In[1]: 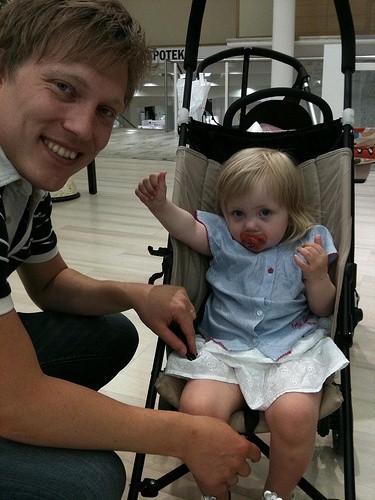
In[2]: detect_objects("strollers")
[127,0,364,500]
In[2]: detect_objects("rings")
[190,306,195,314]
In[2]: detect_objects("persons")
[0,0,262,500]
[134,149,351,500]
